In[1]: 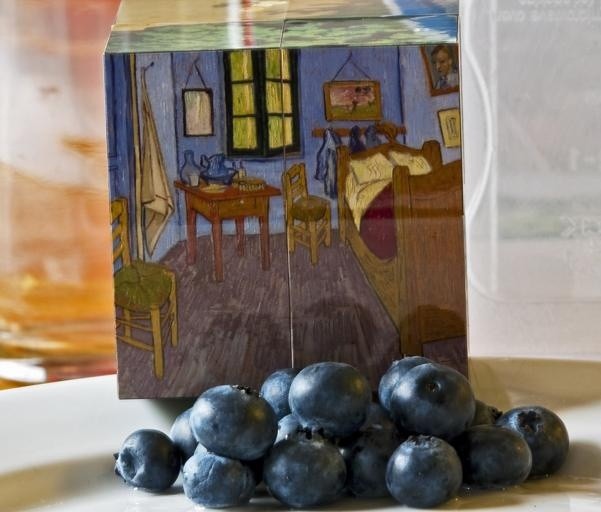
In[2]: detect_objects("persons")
[431,44,459,90]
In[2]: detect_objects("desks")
[2,356,601,512]
